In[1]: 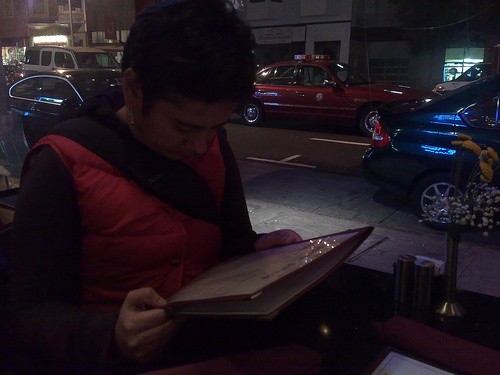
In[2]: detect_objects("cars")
[432,62,494,99]
[0,72,121,129]
[358,72,499,231]
[230,55,422,138]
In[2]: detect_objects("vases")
[425,221,480,317]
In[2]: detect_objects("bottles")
[395,253,416,304]
[414,260,434,309]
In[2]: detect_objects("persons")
[8,0,304,375]
[86,57,93,65]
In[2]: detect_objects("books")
[163,227,374,319]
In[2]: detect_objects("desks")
[139,262,500,375]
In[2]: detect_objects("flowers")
[418,131,500,241]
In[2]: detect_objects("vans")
[23,46,121,79]
